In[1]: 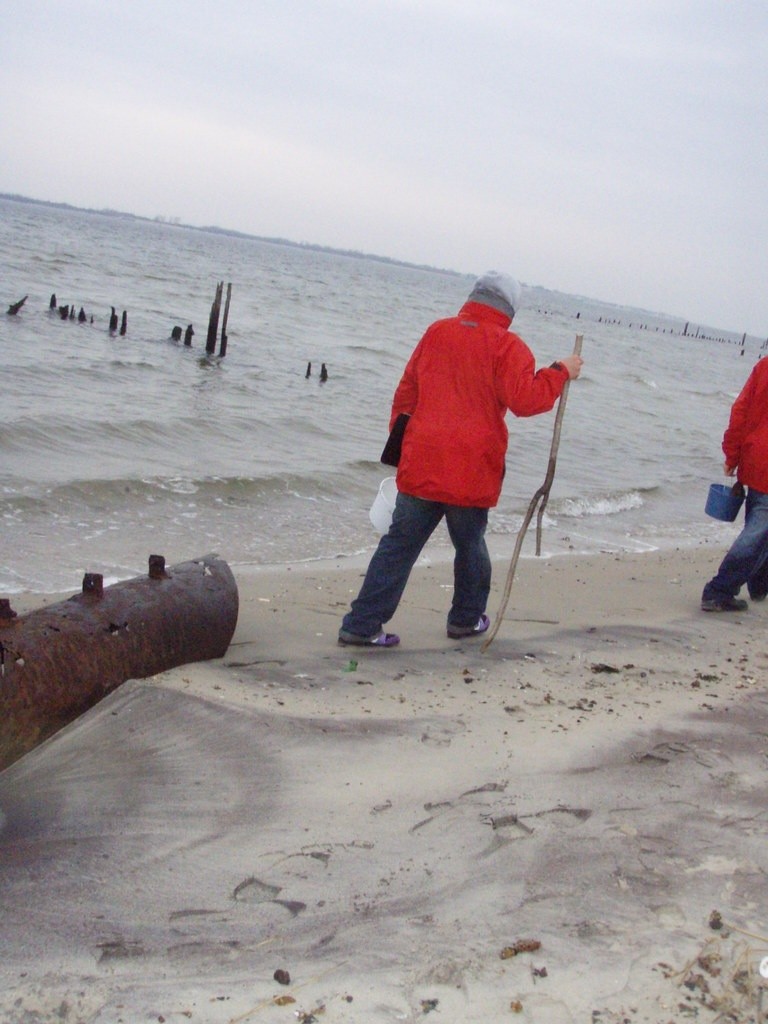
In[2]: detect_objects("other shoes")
[338,627,400,647]
[701,595,748,613]
[751,590,767,601]
[447,614,490,640]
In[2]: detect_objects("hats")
[466,270,522,313]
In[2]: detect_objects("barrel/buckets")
[369,475,398,533]
[704,471,746,523]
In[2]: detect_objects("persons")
[700,355,768,612]
[339,272,584,646]
